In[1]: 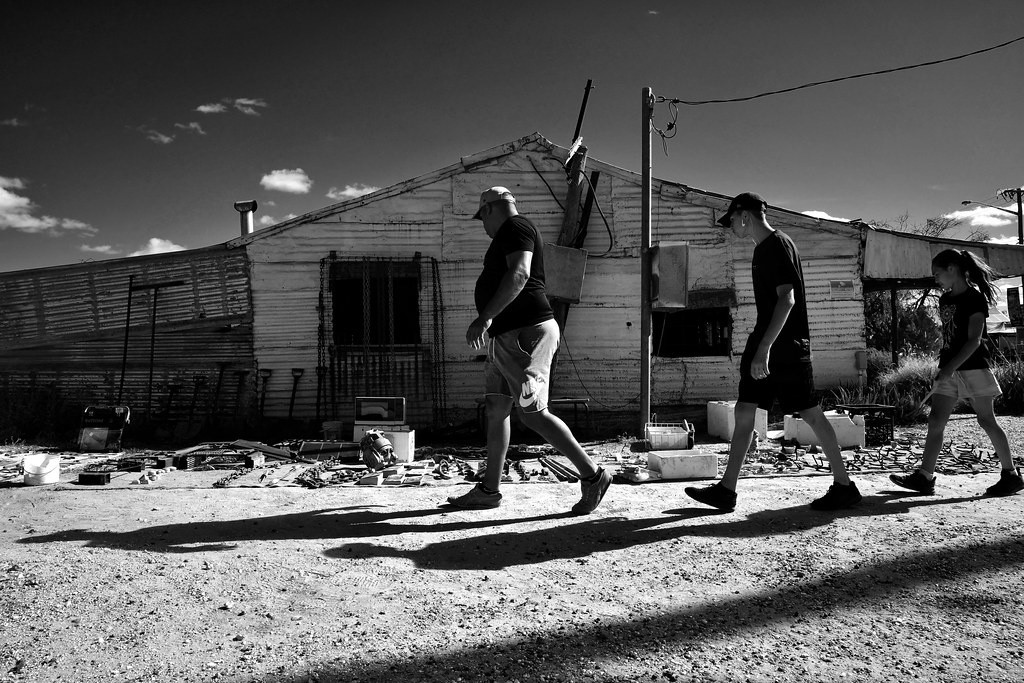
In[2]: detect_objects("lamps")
[235,199,256,236]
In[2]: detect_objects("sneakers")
[889,469,936,494]
[684,483,737,511]
[572,469,613,516]
[447,483,502,508]
[986,468,1024,496]
[809,481,862,511]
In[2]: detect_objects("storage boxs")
[646,426,689,449]
[354,425,416,463]
[707,401,767,442]
[648,450,718,480]
[835,403,897,446]
[783,413,865,448]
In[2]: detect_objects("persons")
[889,248,1024,496]
[447,185,613,514]
[684,191,863,512]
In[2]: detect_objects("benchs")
[475,398,592,438]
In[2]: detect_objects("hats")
[471,186,517,220]
[717,192,768,228]
